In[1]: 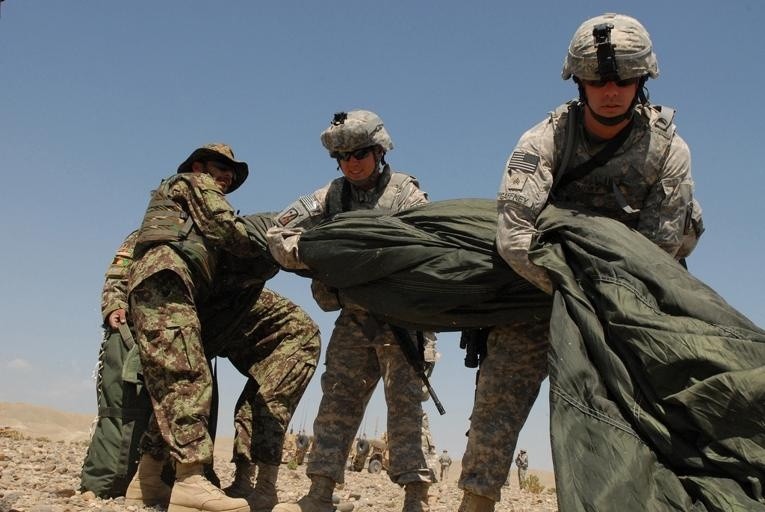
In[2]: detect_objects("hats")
[176,143,249,194]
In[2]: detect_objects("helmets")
[562,12,660,79]
[320,109,394,158]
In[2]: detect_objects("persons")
[514,447,529,489]
[74,228,156,500]
[209,284,323,509]
[456,10,708,511]
[263,109,441,510]
[120,142,254,510]
[437,448,453,483]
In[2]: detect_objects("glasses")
[334,145,374,162]
[582,78,638,88]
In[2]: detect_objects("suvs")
[351,431,389,474]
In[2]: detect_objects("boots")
[125,453,337,512]
[402,484,430,510]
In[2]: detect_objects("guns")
[386,325,448,415]
[460,328,490,436]
